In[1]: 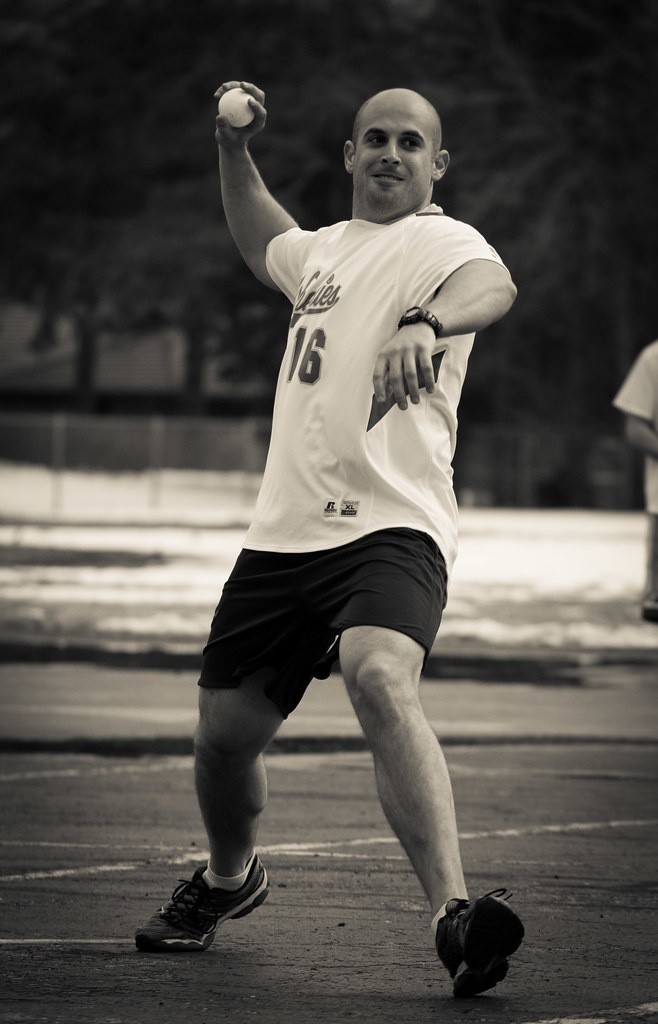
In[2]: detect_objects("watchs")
[399,306,445,336]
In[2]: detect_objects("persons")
[132,79,527,1000]
[612,338,658,624]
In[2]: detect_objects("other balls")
[217,85,259,128]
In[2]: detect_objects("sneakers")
[134,853,270,957]
[435,887,526,1002]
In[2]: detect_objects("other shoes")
[643,608,658,622]
